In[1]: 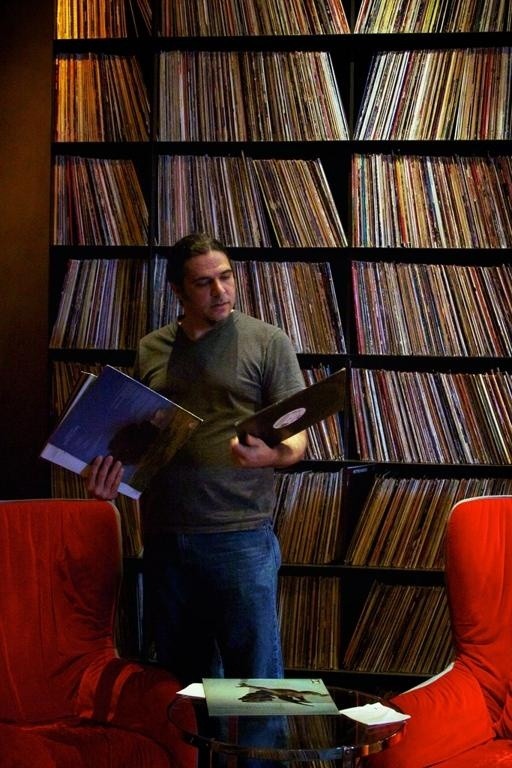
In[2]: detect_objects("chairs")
[371,494,512,768]
[1,491,202,766]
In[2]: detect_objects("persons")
[85,233,307,678]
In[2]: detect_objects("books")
[49,154,512,357]
[56,1,512,140]
[235,363,512,676]
[202,686,388,765]
[202,679,340,716]
[42,362,203,661]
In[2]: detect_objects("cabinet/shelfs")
[50,1,512,686]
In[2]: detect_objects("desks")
[197,671,343,768]
